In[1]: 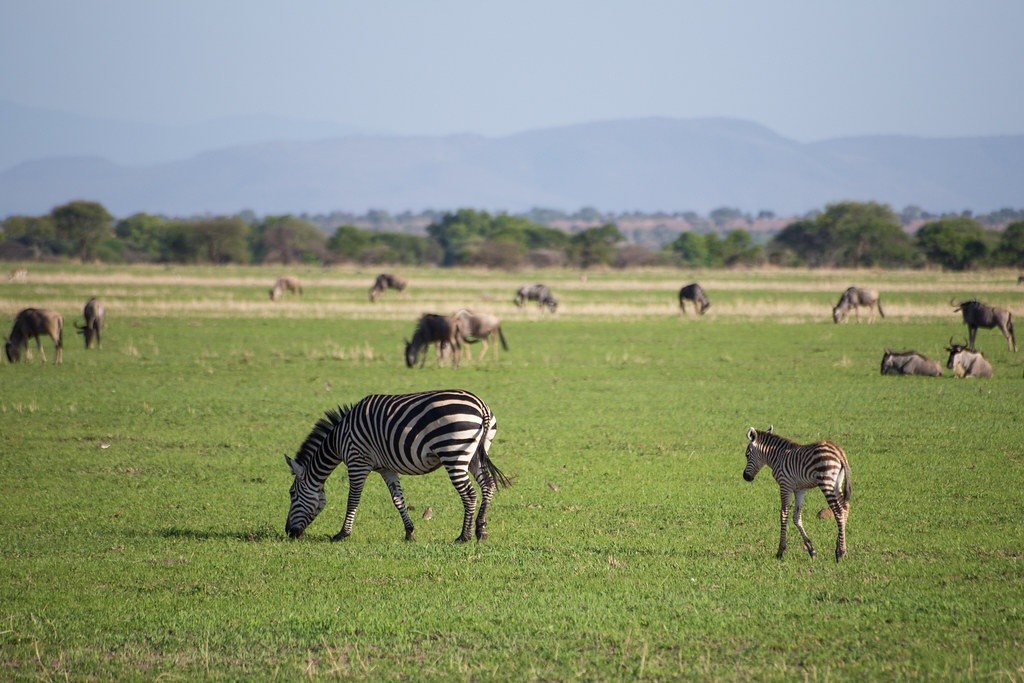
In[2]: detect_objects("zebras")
[743,425,853,563]
[833,286,885,324]
[282,389,514,545]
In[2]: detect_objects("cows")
[269,278,303,303]
[514,283,557,318]
[405,307,509,370]
[368,273,409,304]
[950,295,1018,352]
[4,308,63,365]
[680,284,711,315]
[881,348,943,377]
[74,298,106,350]
[943,335,993,378]
